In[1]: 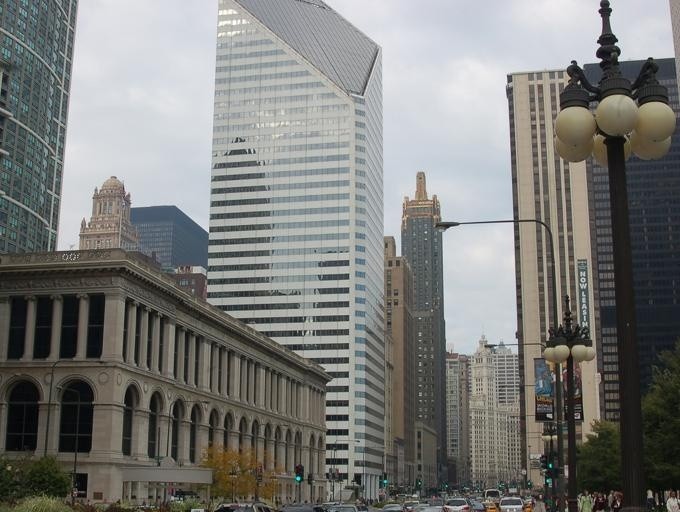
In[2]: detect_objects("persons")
[76,499,91,507]
[532,488,680,511]
[356,496,373,511]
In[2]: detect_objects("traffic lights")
[295,464,304,483]
[419,479,422,486]
[383,472,389,485]
[539,456,548,469]
[547,456,553,478]
[527,480,532,489]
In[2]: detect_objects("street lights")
[228,466,239,503]
[483,340,566,512]
[330,447,337,502]
[333,439,361,501]
[428,218,567,512]
[540,422,559,511]
[546,0,679,512]
[53,385,83,508]
[165,399,212,457]
[540,292,598,512]
